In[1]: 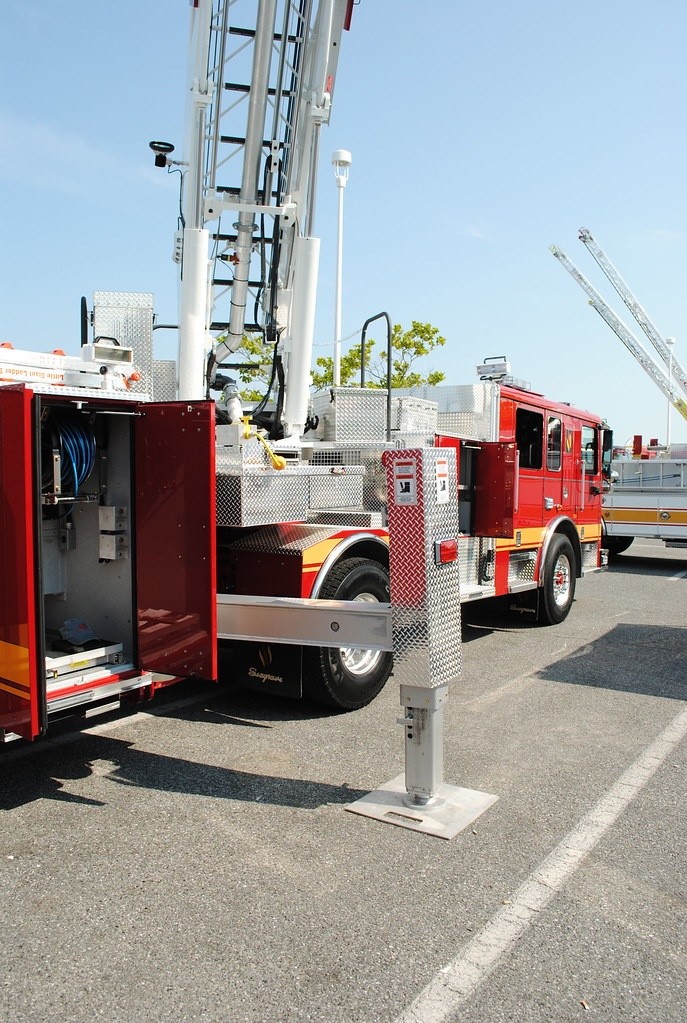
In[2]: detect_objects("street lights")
[331,148,353,388]
[667,336,675,445]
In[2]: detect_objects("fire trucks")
[1,0,615,841]
[548,226,687,557]
